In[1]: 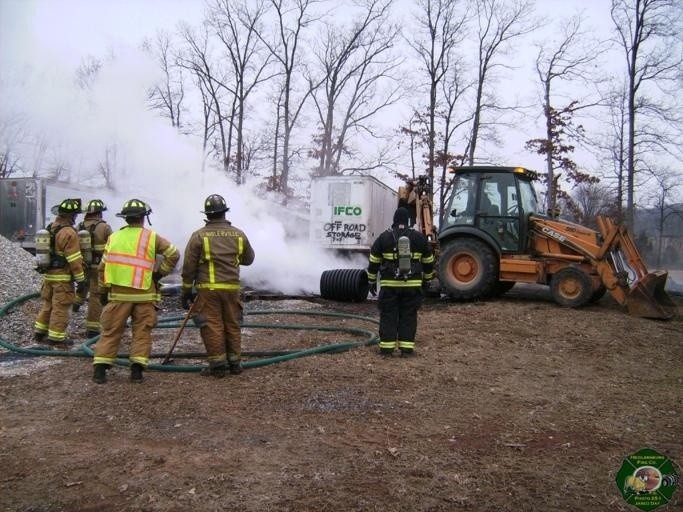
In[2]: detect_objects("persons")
[91,198,180,383]
[72,200,113,338]
[367,208,435,358]
[34,199,85,345]
[181,195,255,378]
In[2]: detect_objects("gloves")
[369,283,377,297]
[153,272,163,283]
[100,294,108,306]
[182,293,194,311]
[76,282,84,294]
[422,282,430,288]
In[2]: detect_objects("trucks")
[309,176,418,266]
[0,177,126,257]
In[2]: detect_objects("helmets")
[50,194,229,218]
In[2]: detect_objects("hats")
[393,207,411,228]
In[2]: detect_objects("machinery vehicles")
[399,165,677,320]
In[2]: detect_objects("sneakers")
[378,350,393,358]
[34,329,243,384]
[400,349,417,358]
